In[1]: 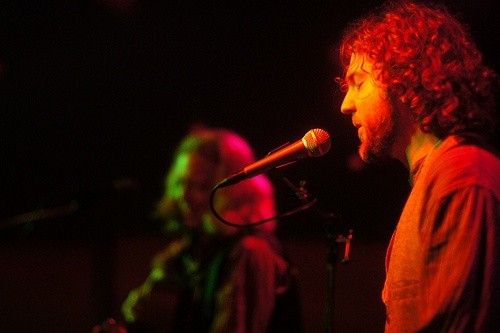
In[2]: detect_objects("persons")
[334,1,500,333]
[112,127,277,333]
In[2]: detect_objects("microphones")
[345,229,353,261]
[218,129,331,189]
